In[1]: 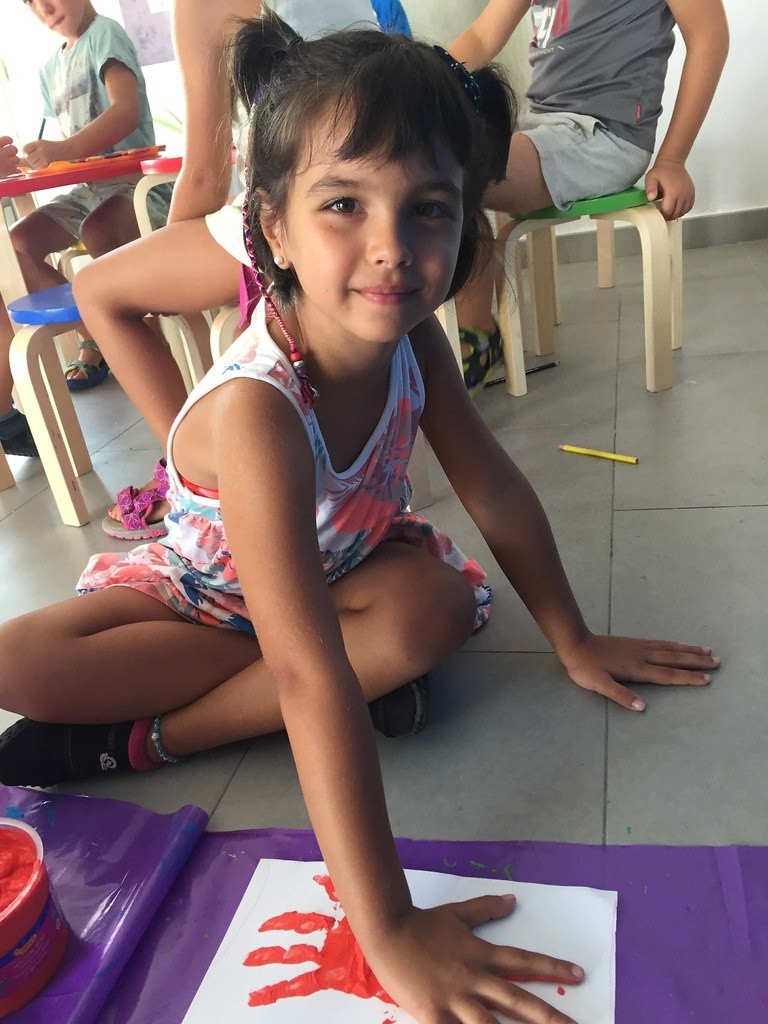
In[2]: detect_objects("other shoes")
[1,711,133,790]
[367,677,428,740]
[0,413,41,457]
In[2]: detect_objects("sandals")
[458,316,505,400]
[63,338,109,390]
[99,457,176,541]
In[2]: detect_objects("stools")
[6,281,464,527]
[494,186,683,392]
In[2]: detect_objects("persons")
[1,136,49,459]
[8,0,176,392]
[437,0,731,400]
[73,0,416,538]
[1,12,727,1024]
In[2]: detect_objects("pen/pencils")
[485,362,561,387]
[38,117,46,139]
[558,444,640,465]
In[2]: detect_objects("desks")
[134,146,238,395]
[0,157,149,477]
